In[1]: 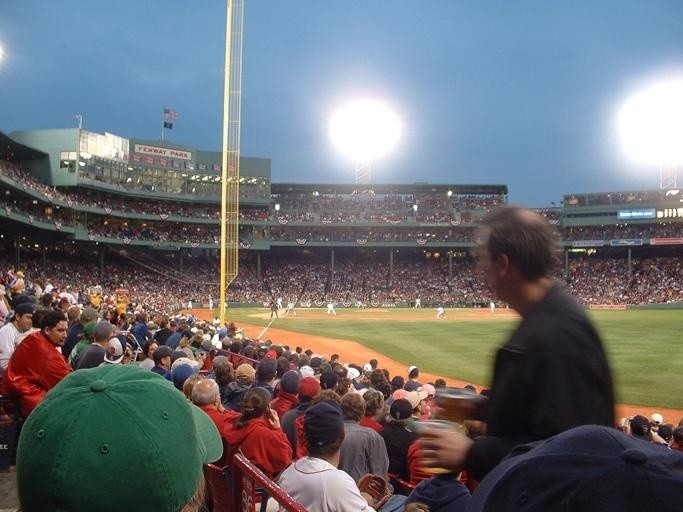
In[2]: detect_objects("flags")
[163,120,174,129]
[165,108,179,120]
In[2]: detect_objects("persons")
[1,130,681,512]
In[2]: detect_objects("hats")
[81,307,127,364]
[181,329,193,339]
[10,303,31,322]
[219,327,227,337]
[461,424,683,512]
[16,363,224,508]
[237,346,435,444]
[650,414,663,425]
[153,345,199,386]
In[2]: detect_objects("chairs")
[216,348,260,372]
[126,333,145,366]
[231,452,310,512]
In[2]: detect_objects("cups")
[436,386,484,428]
[413,418,458,476]
